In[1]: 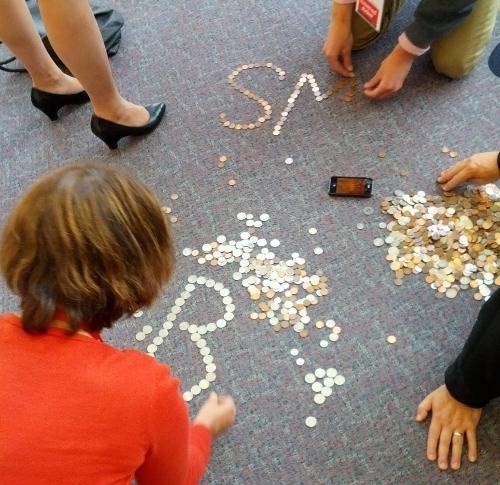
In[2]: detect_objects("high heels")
[31,87,91,120]
[90,103,166,150]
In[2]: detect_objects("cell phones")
[326,175,374,199]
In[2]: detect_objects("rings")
[452,432,464,437]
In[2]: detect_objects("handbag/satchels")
[22,0,124,64]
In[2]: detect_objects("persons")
[0,164,236,485]
[322,0,500,99]
[416,42,500,470]
[1,0,164,149]
